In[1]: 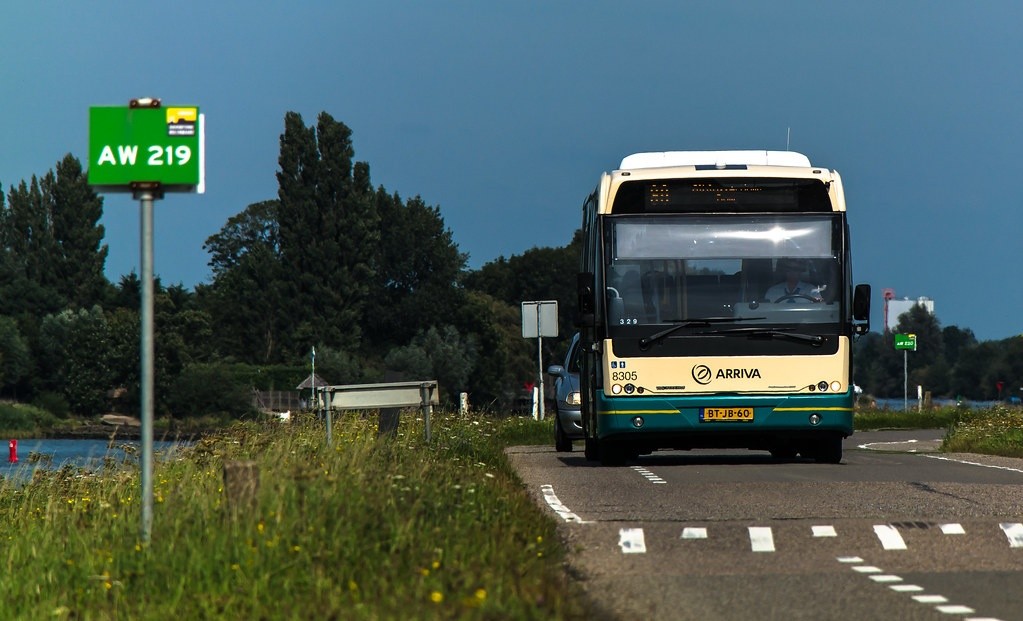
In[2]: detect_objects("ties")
[785,287,800,303]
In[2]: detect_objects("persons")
[765,252,825,305]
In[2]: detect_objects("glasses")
[784,264,806,273]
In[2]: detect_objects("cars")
[547,332,584,453]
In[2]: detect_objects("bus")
[576,151,871,466]
